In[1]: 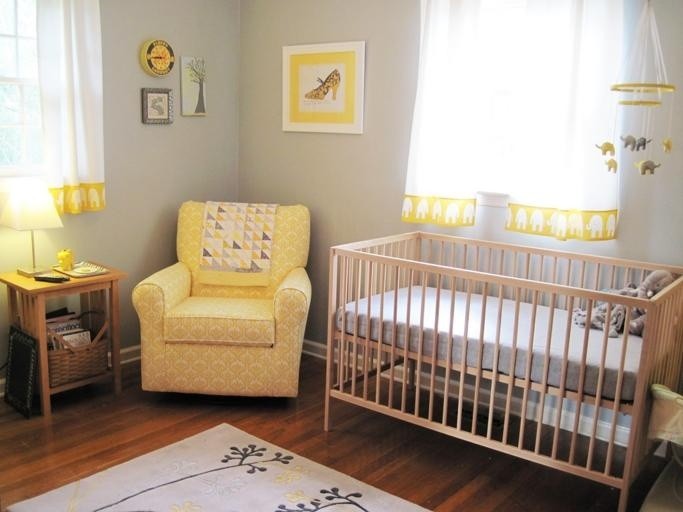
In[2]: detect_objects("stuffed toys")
[593,133,673,176]
[572,283,629,338]
[57,248,74,271]
[616,269,675,334]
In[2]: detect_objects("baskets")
[47,333,108,388]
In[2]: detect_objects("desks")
[1,261,127,417]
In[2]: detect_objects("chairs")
[130,200,314,398]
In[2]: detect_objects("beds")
[323,231,682,512]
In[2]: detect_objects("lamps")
[0,175,65,280]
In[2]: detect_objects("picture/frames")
[4,323,37,419]
[141,89,174,125]
[282,40,366,136]
[180,55,207,116]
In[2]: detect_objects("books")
[42,307,92,351]
[52,260,108,279]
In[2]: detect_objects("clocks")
[140,39,175,77]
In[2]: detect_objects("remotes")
[34,275,69,283]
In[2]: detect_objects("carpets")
[0,420,431,511]
